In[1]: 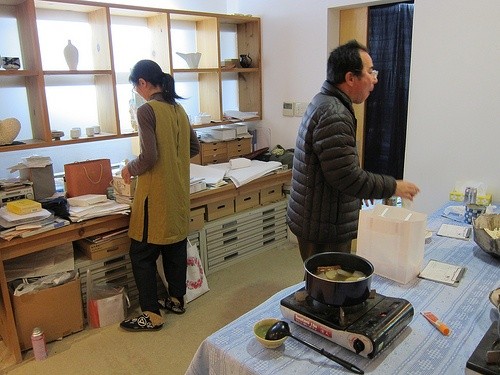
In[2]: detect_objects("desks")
[185,194,500,375]
[0,166,296,363]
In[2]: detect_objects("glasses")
[353,68,379,80]
[131,83,138,94]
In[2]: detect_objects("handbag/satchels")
[161,237,210,304]
[86,268,131,328]
[356,205,426,284]
[64,158,114,198]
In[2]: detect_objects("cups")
[70,127,94,139]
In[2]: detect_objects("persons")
[285,41,420,283]
[119,60,201,332]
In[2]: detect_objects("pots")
[303,252,374,306]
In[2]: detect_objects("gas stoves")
[279,284,414,360]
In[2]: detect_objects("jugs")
[239,54,253,68]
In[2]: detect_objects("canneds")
[464,187,477,206]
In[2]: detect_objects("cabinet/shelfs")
[0,0,262,152]
[191,134,252,165]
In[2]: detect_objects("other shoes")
[158,295,186,315]
[120,309,165,332]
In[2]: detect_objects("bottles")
[64,40,79,70]
[31,327,49,361]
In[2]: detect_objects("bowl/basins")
[253,318,291,349]
[2,57,20,71]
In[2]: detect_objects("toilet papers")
[194,115,210,125]
[229,159,251,169]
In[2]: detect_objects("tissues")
[113,160,137,196]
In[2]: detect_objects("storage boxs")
[111,175,136,197]
[6,271,84,352]
[260,183,283,205]
[190,207,206,231]
[19,164,56,201]
[78,236,130,260]
[234,190,260,212]
[7,199,41,214]
[205,197,234,221]
